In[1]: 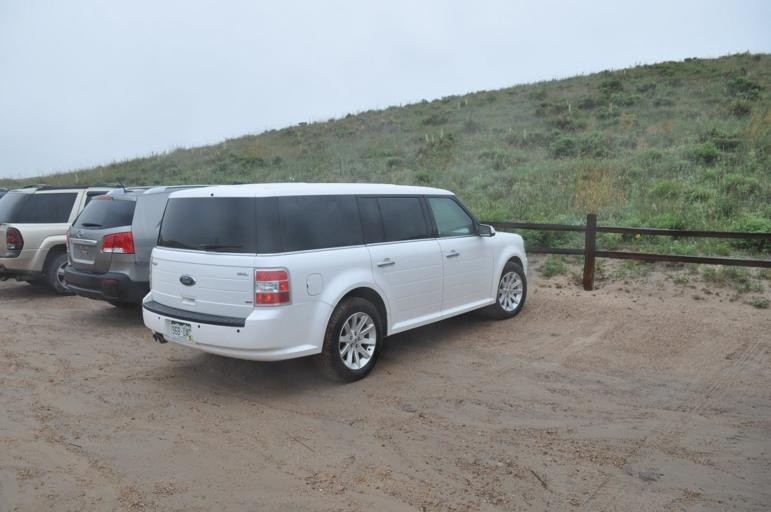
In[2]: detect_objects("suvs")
[53,184,208,309]
[1,185,88,296]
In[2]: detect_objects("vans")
[137,181,532,383]
